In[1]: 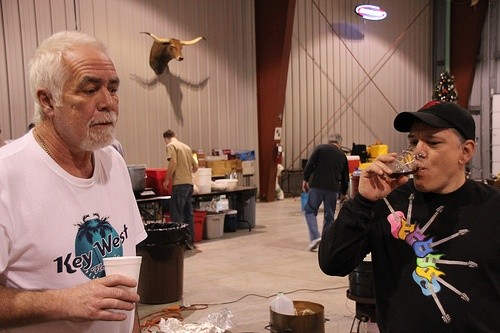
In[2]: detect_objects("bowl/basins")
[215,180,238,191]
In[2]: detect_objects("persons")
[0,30,149,333]
[163,130,199,251]
[318,100,500,333]
[302,132,349,250]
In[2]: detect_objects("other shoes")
[310,237,322,249]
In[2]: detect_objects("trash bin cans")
[265,300,329,333]
[136,223,190,304]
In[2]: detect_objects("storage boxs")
[346,144,388,171]
[125,149,256,242]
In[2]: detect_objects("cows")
[140,31,208,75]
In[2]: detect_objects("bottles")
[196,150,206,167]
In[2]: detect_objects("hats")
[394,100,475,144]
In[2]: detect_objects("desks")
[283,168,303,198]
[135,185,258,245]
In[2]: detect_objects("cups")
[222,148,231,160]
[102,256,142,314]
[212,149,219,156]
[383,146,427,180]
[300,192,309,211]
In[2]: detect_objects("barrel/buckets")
[193,167,212,194]
[193,211,207,242]
[346,155,360,173]
[371,144,388,163]
[127,163,147,199]
[265,299,330,333]
[145,169,172,196]
